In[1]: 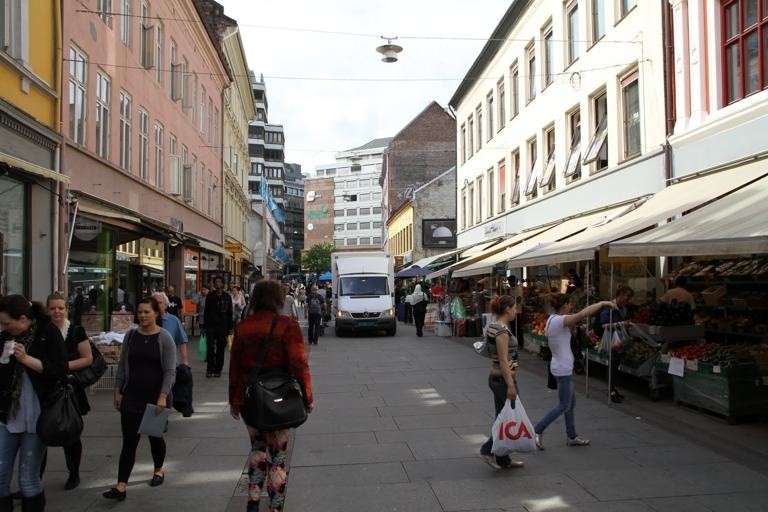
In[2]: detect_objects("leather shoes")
[102,487,126,501]
[151,469,165,486]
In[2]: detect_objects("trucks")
[331,249,398,337]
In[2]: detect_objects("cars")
[241,294,298,324]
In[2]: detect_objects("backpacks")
[307,294,323,316]
[593,305,605,339]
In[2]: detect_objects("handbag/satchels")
[472,340,493,358]
[547,360,558,390]
[35,382,84,447]
[72,323,109,390]
[413,301,427,314]
[239,374,309,432]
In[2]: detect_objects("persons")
[659,276,696,310]
[152,277,334,434]
[103,297,177,502]
[507,276,523,350]
[40,292,106,489]
[395,284,429,337]
[71,285,130,320]
[477,295,524,471]
[229,282,313,512]
[534,294,620,451]
[594,286,635,404]
[0,294,67,512]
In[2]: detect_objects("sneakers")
[480,455,503,470]
[535,432,545,451]
[607,389,625,404]
[63,474,81,490]
[566,435,591,447]
[511,460,525,467]
